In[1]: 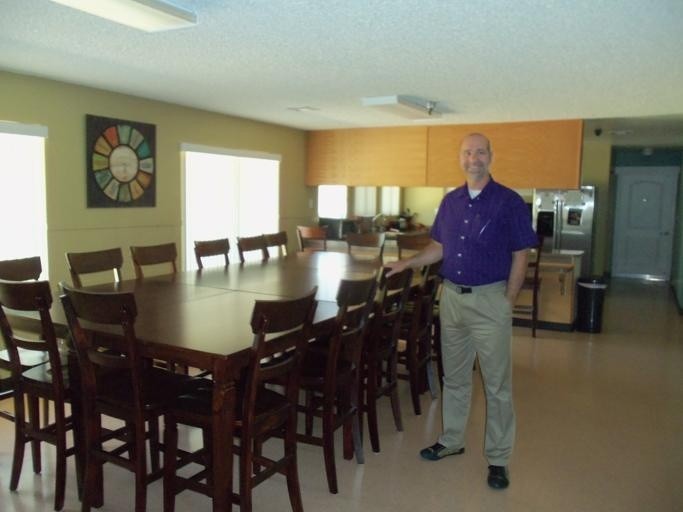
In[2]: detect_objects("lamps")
[363,96,442,121]
[49,1,198,34]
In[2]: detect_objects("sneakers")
[421,443,464,460]
[489,465,511,489]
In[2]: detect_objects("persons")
[384,133,537,490]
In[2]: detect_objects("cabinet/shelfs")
[512,263,576,331]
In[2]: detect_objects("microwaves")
[322,217,362,238]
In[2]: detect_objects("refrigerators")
[532,185,597,277]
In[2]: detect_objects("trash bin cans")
[578,274,608,333]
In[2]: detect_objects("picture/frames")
[85,112,157,208]
